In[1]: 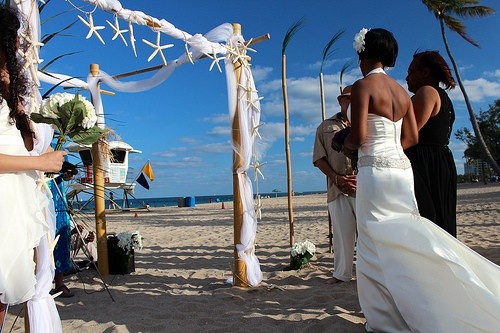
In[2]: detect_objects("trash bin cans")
[186,196,195,207]
[178,197,186,207]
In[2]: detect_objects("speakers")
[107,236,136,274]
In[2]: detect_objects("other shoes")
[324,277,340,285]
[363,321,376,333]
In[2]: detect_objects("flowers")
[28,92,106,179]
[286,240,316,271]
[330,126,357,171]
[117,231,142,255]
[353,27,372,52]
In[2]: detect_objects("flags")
[143,161,154,181]
[137,172,149,190]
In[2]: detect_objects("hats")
[337,85,352,101]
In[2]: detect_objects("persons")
[343,28,500,333]
[46,172,74,297]
[313,85,357,284]
[109,192,115,209]
[68,201,97,262]
[0,3,67,333]
[406,51,457,237]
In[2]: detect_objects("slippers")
[50,285,74,297]
